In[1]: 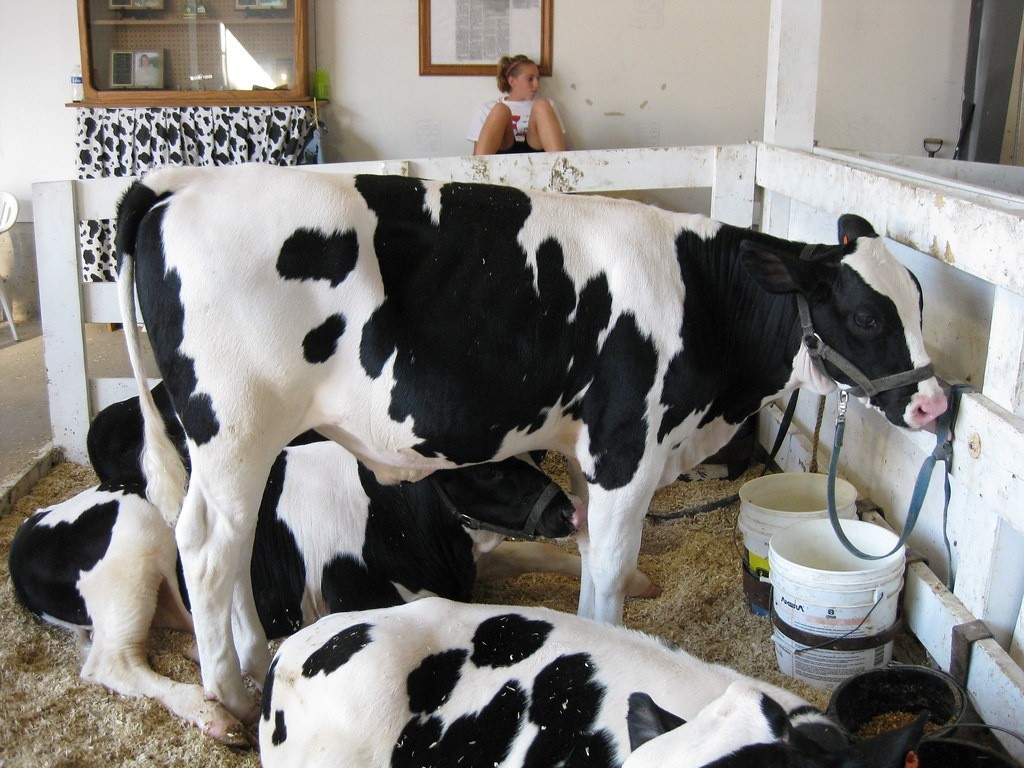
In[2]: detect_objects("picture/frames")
[110,48,164,88]
[108,0,164,10]
[233,0,287,10]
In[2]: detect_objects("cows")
[258,596,926,768]
[9,163,947,744]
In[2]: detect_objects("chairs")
[0,192,18,341]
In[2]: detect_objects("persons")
[135,54,158,84]
[466,54,567,157]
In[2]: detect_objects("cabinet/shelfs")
[76,0,312,103]
